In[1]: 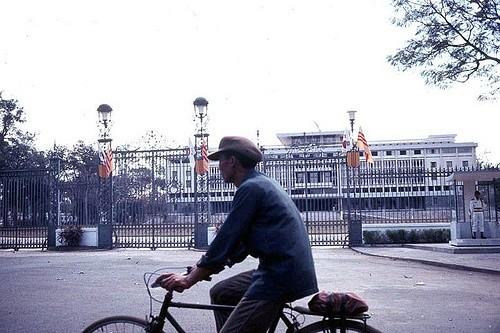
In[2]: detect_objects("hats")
[474,191,481,195]
[207,136,263,163]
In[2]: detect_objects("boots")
[481,232,487,239]
[473,232,476,239]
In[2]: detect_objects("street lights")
[346,110,365,248]
[95,105,117,250]
[193,97,216,252]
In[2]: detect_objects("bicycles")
[82,266,384,333]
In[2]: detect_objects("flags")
[155,135,320,333]
[97,140,113,176]
[343,125,373,167]
[188,138,210,176]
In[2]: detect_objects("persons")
[468,191,488,239]
